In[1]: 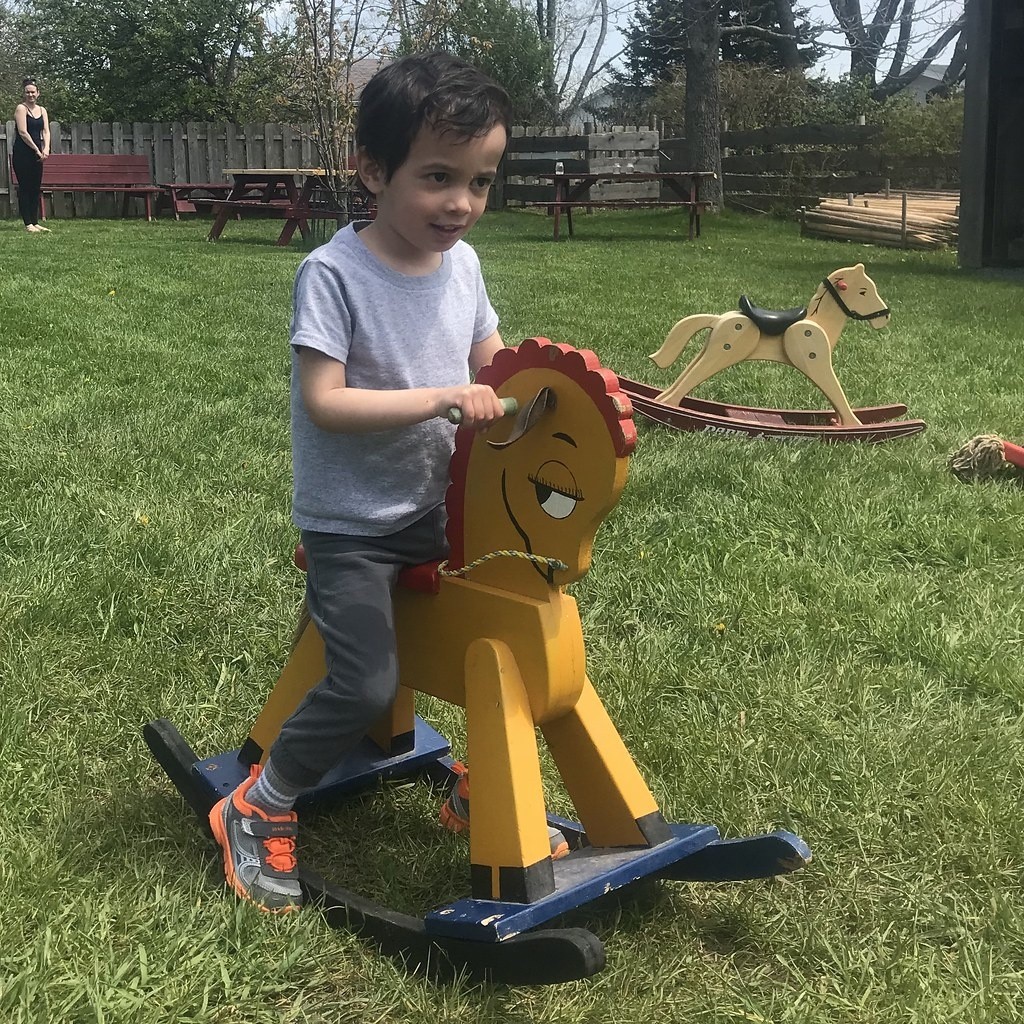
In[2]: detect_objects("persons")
[13,76,51,232]
[208,52,513,916]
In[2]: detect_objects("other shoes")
[35,224,52,232]
[26,224,40,232]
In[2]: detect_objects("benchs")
[158,155,378,246]
[526,201,721,242]
[9,154,166,219]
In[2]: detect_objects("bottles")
[556,161,564,175]
[612,163,621,174]
[625,163,634,174]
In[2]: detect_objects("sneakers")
[440,764,568,859]
[209,764,306,913]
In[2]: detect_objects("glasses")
[23,81,36,88]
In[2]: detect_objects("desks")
[539,171,719,242]
[207,168,358,246]
